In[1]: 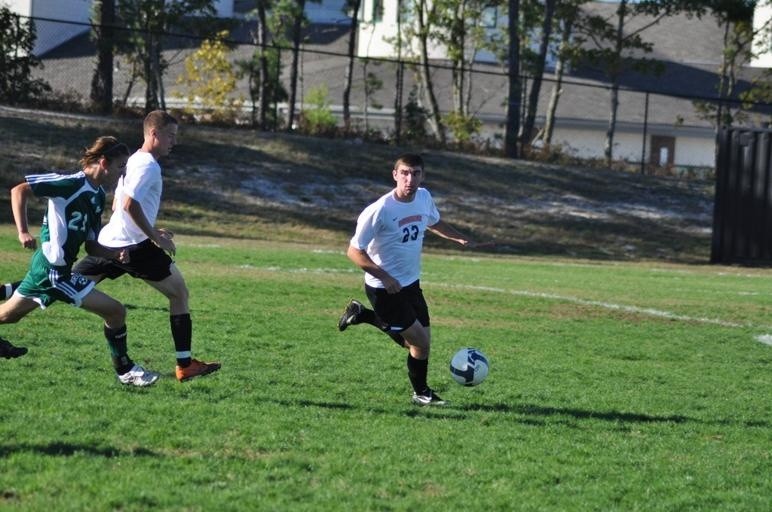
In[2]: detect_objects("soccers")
[451,349,490,387]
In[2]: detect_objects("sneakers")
[175,359,221,383]
[339,299,363,332]
[0,338,28,359]
[117,365,159,387]
[413,388,448,405]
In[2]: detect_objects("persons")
[0,110,221,383]
[0,135,161,387]
[338,153,495,406]
